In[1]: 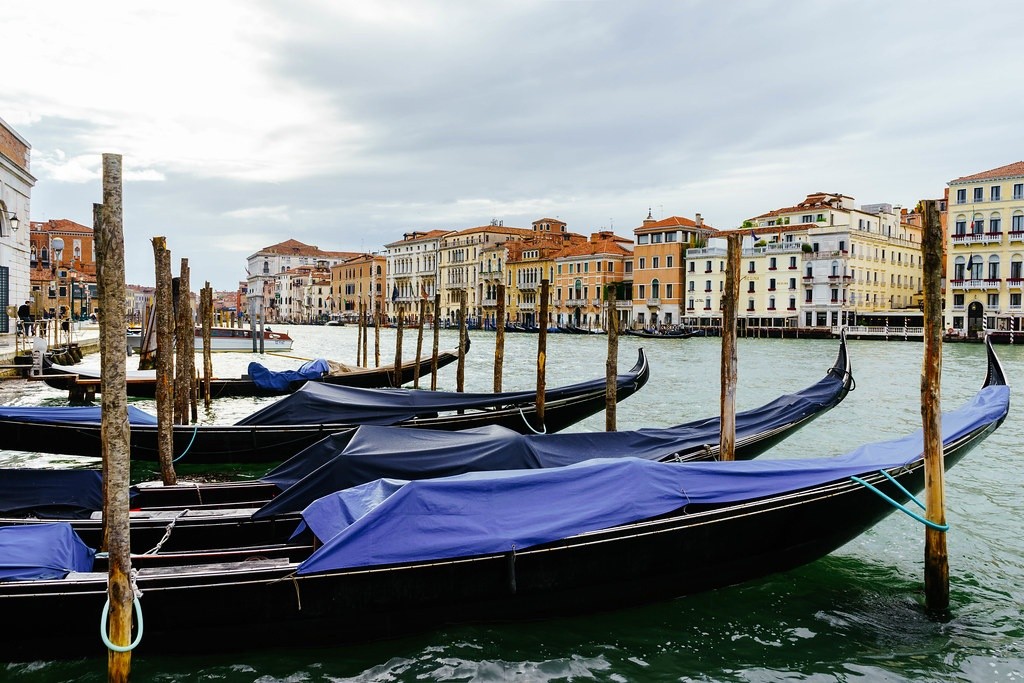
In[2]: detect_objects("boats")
[490,323,595,334]
[624,326,704,338]
[42,325,471,394]
[1,340,1009,660]
[124,326,294,352]
[0,346,651,465]
[2,329,856,528]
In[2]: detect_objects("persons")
[18,300,35,337]
[997,324,1007,331]
[48,311,54,329]
[39,308,48,335]
[61,317,71,344]
[636,320,691,338]
[75,313,79,323]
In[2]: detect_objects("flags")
[391,285,398,303]
[420,287,429,298]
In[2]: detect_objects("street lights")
[84,282,89,320]
[51,237,64,350]
[68,268,77,333]
[78,280,85,321]
